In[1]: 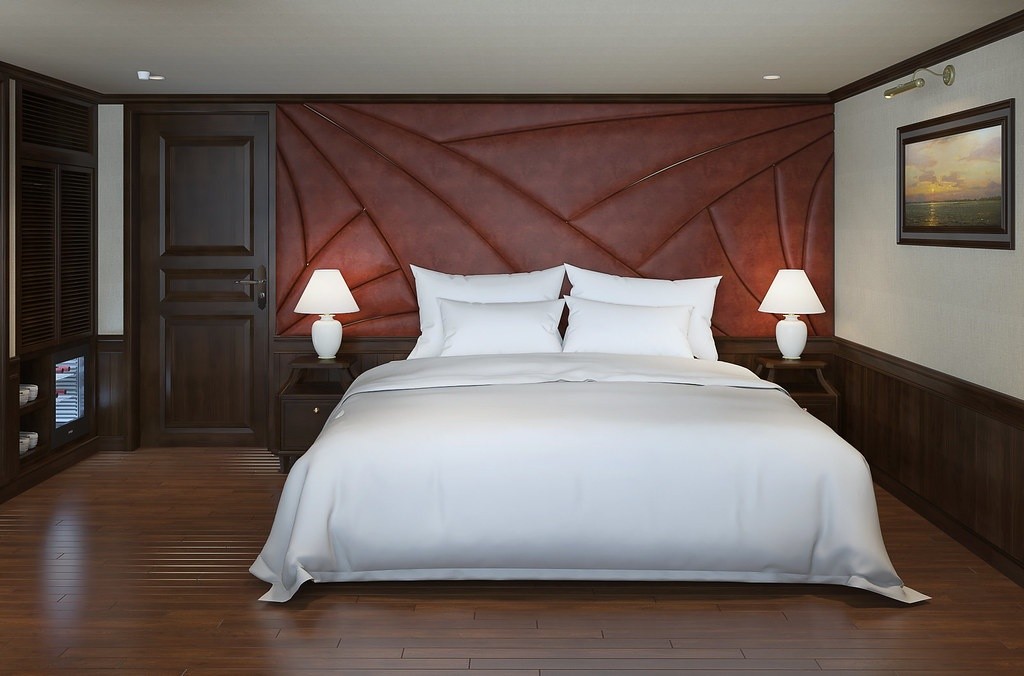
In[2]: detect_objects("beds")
[247,353,934,603]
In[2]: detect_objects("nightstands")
[277,357,359,474]
[752,358,840,438]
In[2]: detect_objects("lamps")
[757,269,826,359]
[293,269,361,360]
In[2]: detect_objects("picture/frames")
[895,97,1015,250]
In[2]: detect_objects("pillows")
[436,297,565,357]
[561,294,695,359]
[563,263,724,360]
[405,263,566,360]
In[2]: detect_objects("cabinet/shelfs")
[0,336,99,505]
[8,78,99,360]
